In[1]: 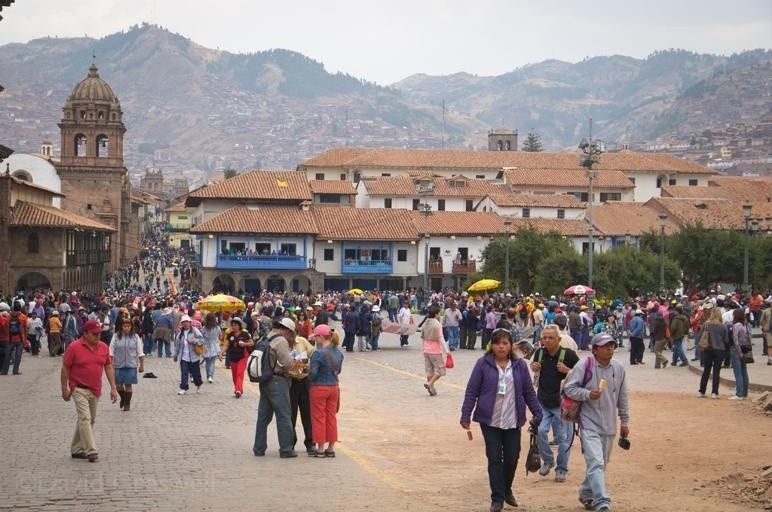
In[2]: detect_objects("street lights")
[579,124,601,295]
[740,196,756,301]
[502,219,513,295]
[420,232,432,299]
[657,214,670,292]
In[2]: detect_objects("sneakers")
[308,446,335,458]
[556,470,567,481]
[635,357,700,368]
[358,345,381,351]
[539,463,555,475]
[179,376,213,395]
[424,381,437,395]
[698,392,743,400]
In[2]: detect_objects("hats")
[591,333,617,346]
[671,292,732,310]
[326,303,336,311]
[163,307,192,322]
[279,318,296,332]
[508,292,588,311]
[84,320,102,334]
[308,324,330,337]
[363,300,381,312]
[306,306,313,311]
[635,309,644,315]
[51,306,86,316]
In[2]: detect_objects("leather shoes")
[505,497,518,506]
[490,502,503,512]
[72,453,88,458]
[280,452,297,457]
[88,454,97,462]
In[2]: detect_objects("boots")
[118,391,124,408]
[124,392,132,411]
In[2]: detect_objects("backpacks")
[9,313,22,334]
[246,334,282,382]
[560,356,594,421]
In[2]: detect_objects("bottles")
[618,438,630,450]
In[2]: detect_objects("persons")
[566,332,631,510]
[430,253,442,268]
[455,250,474,265]
[222,285,424,395]
[253,317,344,459]
[420,283,772,400]
[345,256,393,267]
[223,246,288,261]
[1,288,110,375]
[108,317,145,412]
[531,324,583,484]
[61,320,117,461]
[102,221,222,397]
[456,328,545,512]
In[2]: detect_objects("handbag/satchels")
[741,345,754,363]
[195,344,205,354]
[698,323,709,351]
[526,435,540,475]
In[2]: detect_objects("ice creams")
[599,379,607,391]
